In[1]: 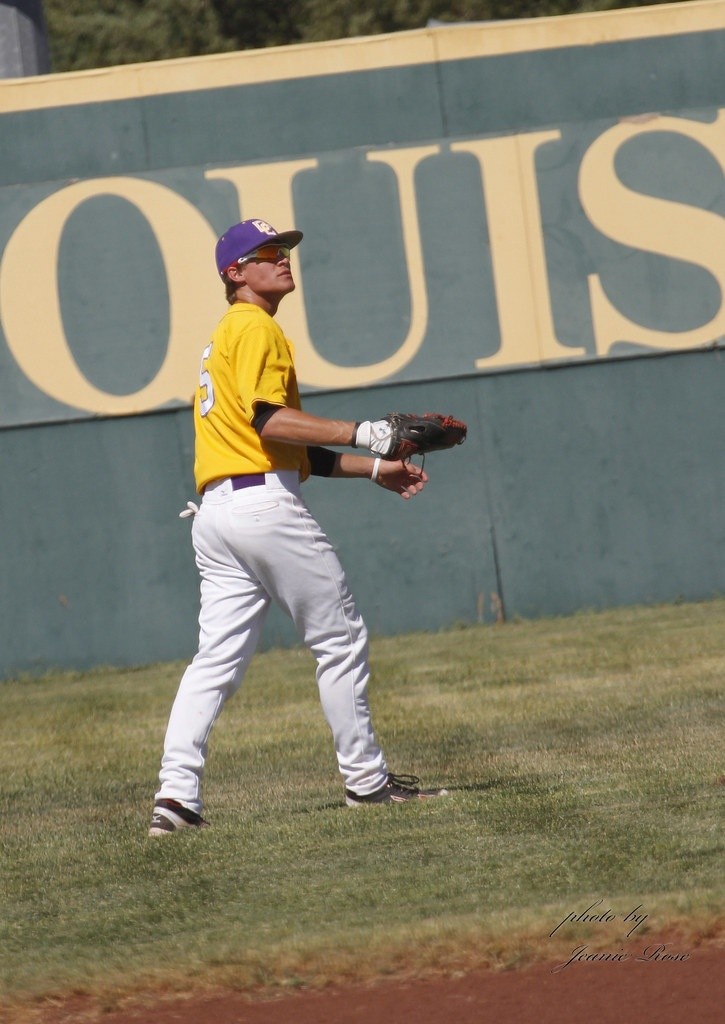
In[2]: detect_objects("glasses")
[221,243,291,276]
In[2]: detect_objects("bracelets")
[370,457,382,484]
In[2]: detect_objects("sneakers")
[345,773,447,808]
[147,798,211,838]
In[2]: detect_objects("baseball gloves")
[356,413,467,476]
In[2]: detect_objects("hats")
[215,219,304,282]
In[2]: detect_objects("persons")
[144,220,464,838]
